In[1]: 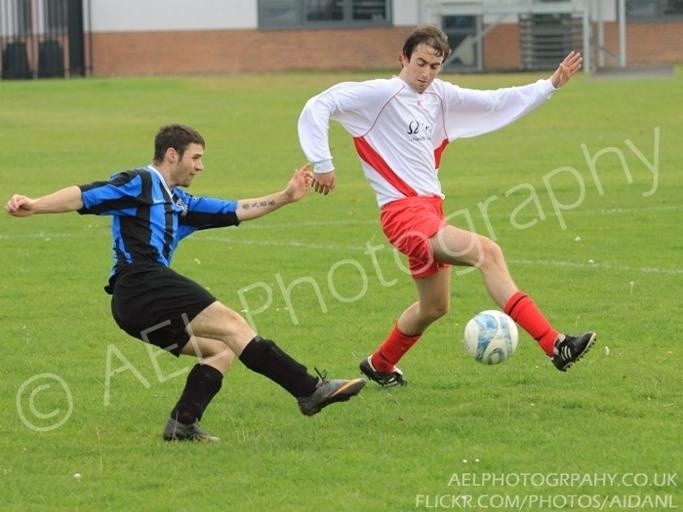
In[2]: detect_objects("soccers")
[464,309,518,364]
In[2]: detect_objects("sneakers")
[549,332,597,372]
[296,356,407,416]
[164,418,220,442]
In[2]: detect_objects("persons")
[4,124,365,444]
[296,21,596,391]
